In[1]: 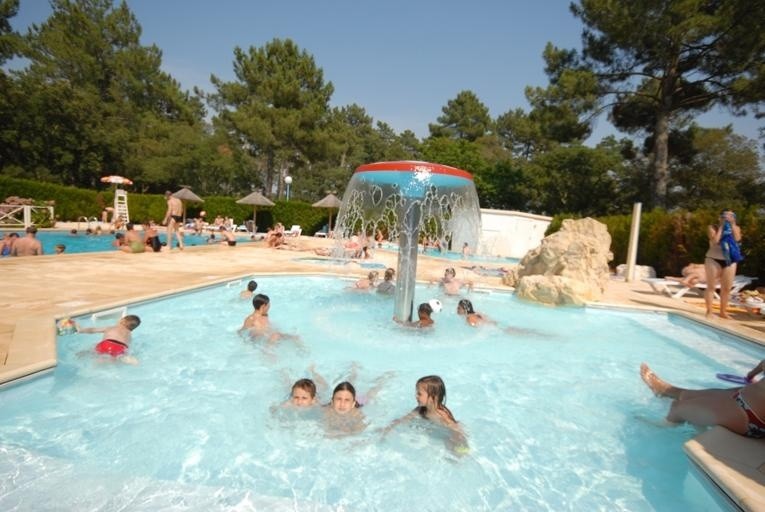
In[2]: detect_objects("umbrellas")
[100,175,133,189]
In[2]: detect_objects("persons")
[250,222,288,250]
[270,363,328,416]
[69,211,166,252]
[322,365,395,439]
[70,315,141,363]
[160,191,237,249]
[242,280,269,328]
[637,362,765,438]
[1,225,65,258]
[705,209,742,318]
[665,262,705,288]
[378,375,470,456]
[312,225,484,328]
[747,359,765,379]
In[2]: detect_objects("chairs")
[177,213,337,240]
[729,288,765,317]
[640,270,759,301]
